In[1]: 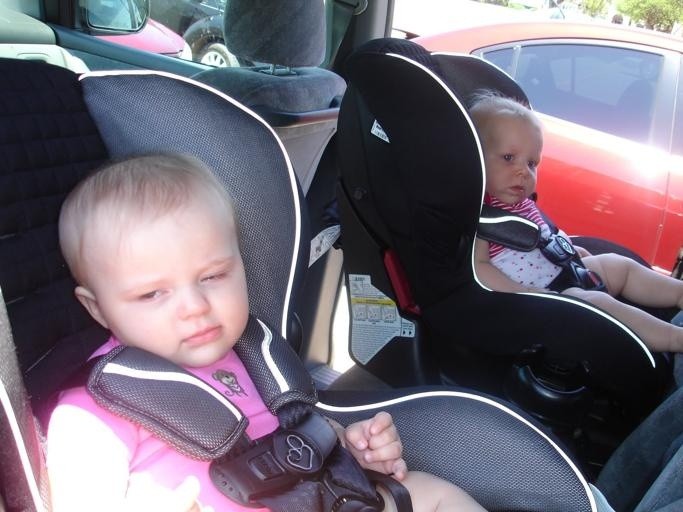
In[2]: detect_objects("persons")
[468,88,682,353]
[43,150,490,512]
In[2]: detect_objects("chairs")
[0,52,616,511]
[179,0,347,208]
[320,37,677,495]
